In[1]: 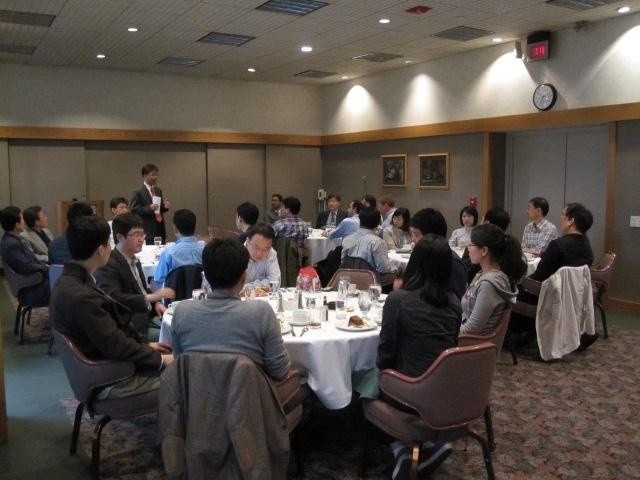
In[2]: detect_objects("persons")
[236,194,594,346]
[375,237,463,479]
[1,197,208,347]
[172,241,291,384]
[50,216,173,399]
[127,164,171,244]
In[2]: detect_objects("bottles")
[321,296,329,321]
[277,291,303,310]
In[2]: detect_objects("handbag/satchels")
[315,246,342,287]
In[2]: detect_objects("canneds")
[269,280,279,298]
[245,285,255,300]
[368,283,382,301]
[338,279,349,299]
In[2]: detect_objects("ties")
[133,261,153,311]
[331,212,335,223]
[150,187,162,223]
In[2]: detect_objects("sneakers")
[515,330,535,348]
[577,332,598,351]
[391,447,413,480]
[418,440,453,474]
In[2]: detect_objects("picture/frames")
[415,152,450,191]
[380,154,408,190]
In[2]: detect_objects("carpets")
[59,319,639,479]
[1,277,52,339]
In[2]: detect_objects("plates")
[337,321,379,332]
[280,319,313,336]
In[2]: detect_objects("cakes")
[348,315,366,328]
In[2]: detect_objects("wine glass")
[154,237,162,254]
[358,283,382,322]
[269,278,281,300]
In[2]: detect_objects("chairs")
[0,223,617,479]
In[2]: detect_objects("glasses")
[127,233,148,238]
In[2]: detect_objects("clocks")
[532,83,557,111]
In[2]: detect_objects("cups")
[335,280,349,317]
[245,287,255,301]
[294,310,311,322]
[449,240,455,250]
